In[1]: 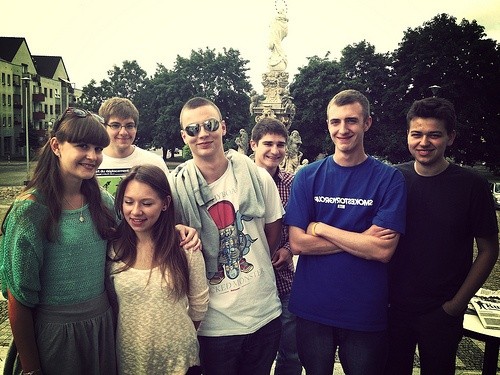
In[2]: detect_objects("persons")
[105,165,211,375]
[282,89,406,375]
[0,107,202,375]
[380,98,498,375]
[249,118,303,375]
[167,97,286,375]
[94,97,170,197]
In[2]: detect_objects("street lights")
[20,77,34,186]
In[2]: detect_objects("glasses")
[54,107,105,133]
[183,119,221,137]
[105,122,136,130]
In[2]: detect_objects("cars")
[488,179,500,210]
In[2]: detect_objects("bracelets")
[311,222,319,237]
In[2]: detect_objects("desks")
[462,311,500,375]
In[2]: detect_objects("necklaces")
[63,191,85,223]
